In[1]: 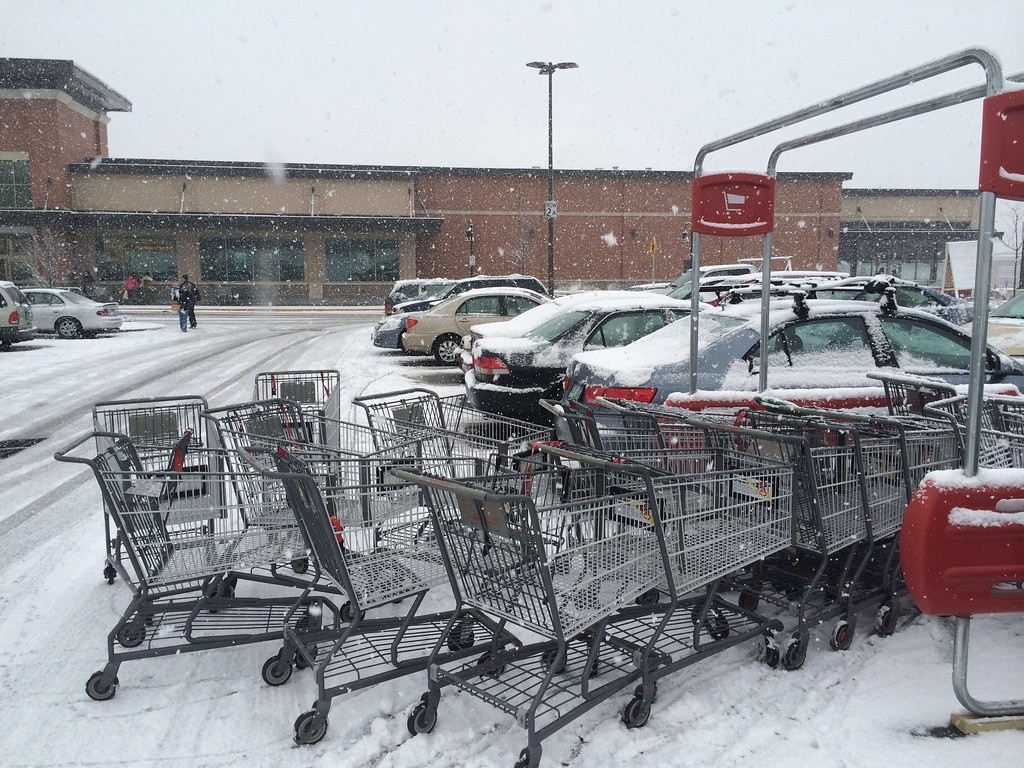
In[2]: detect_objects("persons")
[874,267,899,278]
[81,271,95,299]
[125,272,153,305]
[173,274,201,332]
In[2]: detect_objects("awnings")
[840,228,978,233]
[0,209,445,220]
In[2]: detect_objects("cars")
[665,270,1024,370]
[372,286,1024,465]
[17,289,121,338]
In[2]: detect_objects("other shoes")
[189,324,197,328]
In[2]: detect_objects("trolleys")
[52,370,1024,767]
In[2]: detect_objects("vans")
[385,278,459,314]
[647,263,757,301]
[0,281,33,349]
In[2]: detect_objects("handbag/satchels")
[172,304,181,312]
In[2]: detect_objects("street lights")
[527,60,578,297]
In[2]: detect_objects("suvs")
[394,275,550,316]
